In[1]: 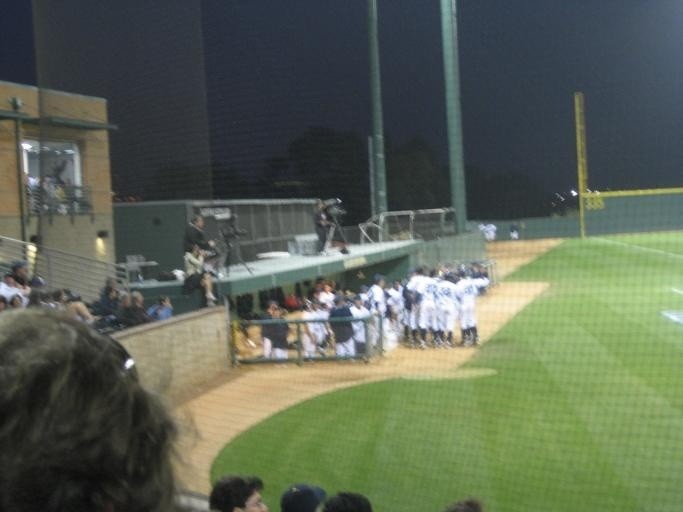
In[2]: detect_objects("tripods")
[224,240,251,276]
[318,220,354,257]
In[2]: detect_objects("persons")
[445,496,483,511]
[185,215,217,251]
[0,258,172,334]
[183,240,218,307]
[0,303,188,512]
[237,259,491,362]
[209,475,268,512]
[321,490,372,512]
